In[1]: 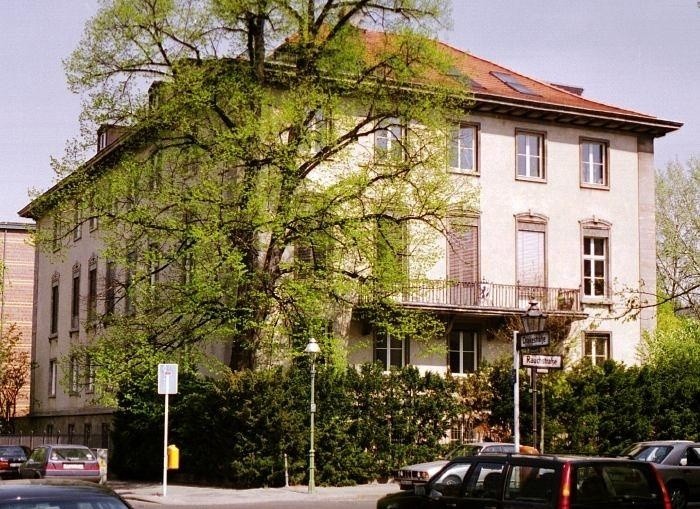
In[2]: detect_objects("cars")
[0,444,133,508]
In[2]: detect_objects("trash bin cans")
[91,448,108,484]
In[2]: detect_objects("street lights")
[520,299,550,456]
[302,337,323,496]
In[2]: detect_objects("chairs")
[483,472,608,500]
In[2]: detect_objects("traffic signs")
[517,331,549,348]
[522,354,563,369]
